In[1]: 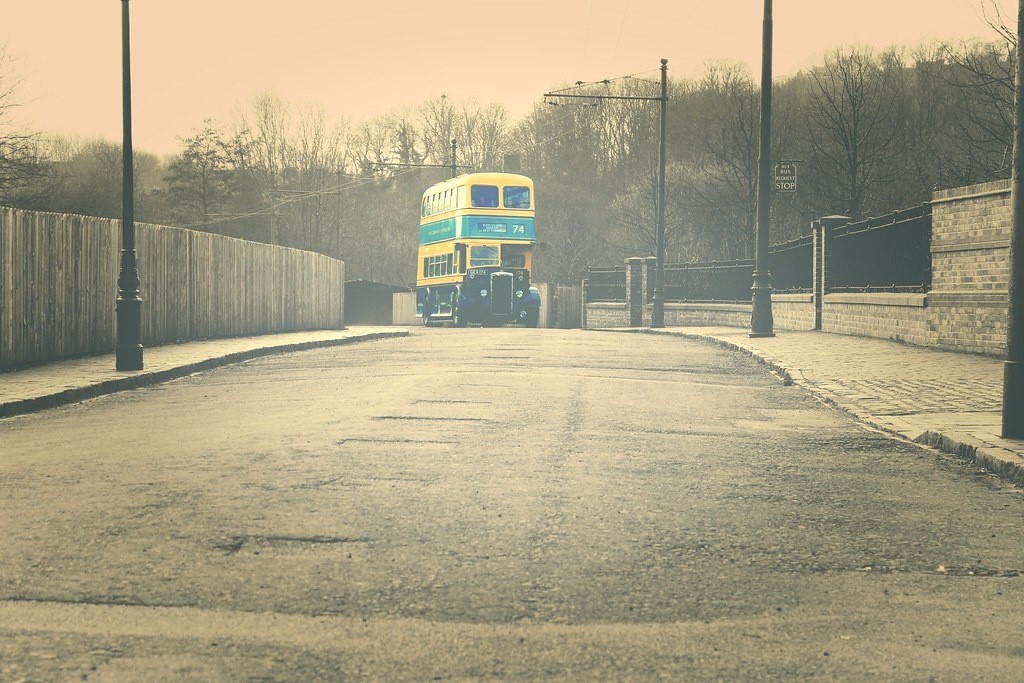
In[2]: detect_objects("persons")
[506,200,513,208]
[476,193,485,207]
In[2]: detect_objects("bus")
[415,171,541,328]
